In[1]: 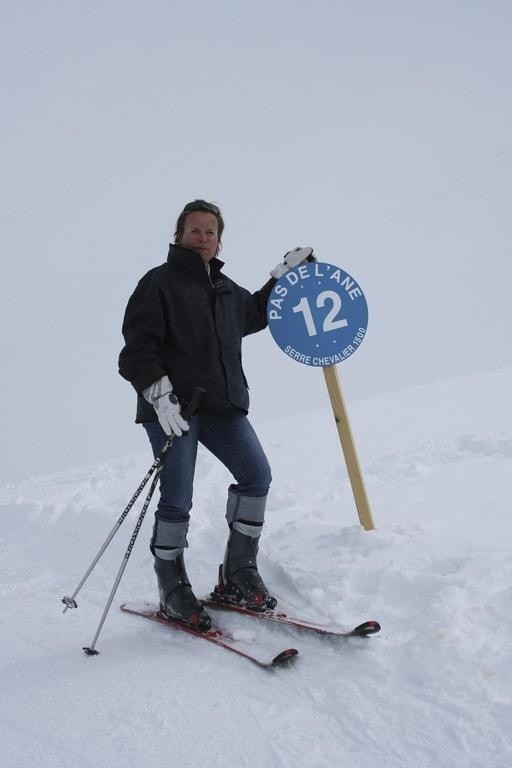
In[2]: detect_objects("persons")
[121,199,312,628]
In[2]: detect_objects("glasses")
[183,202,219,216]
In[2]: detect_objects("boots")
[223,521,277,610]
[153,547,212,632]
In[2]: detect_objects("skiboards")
[118,593,380,667]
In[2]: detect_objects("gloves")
[141,374,191,438]
[269,246,316,280]
